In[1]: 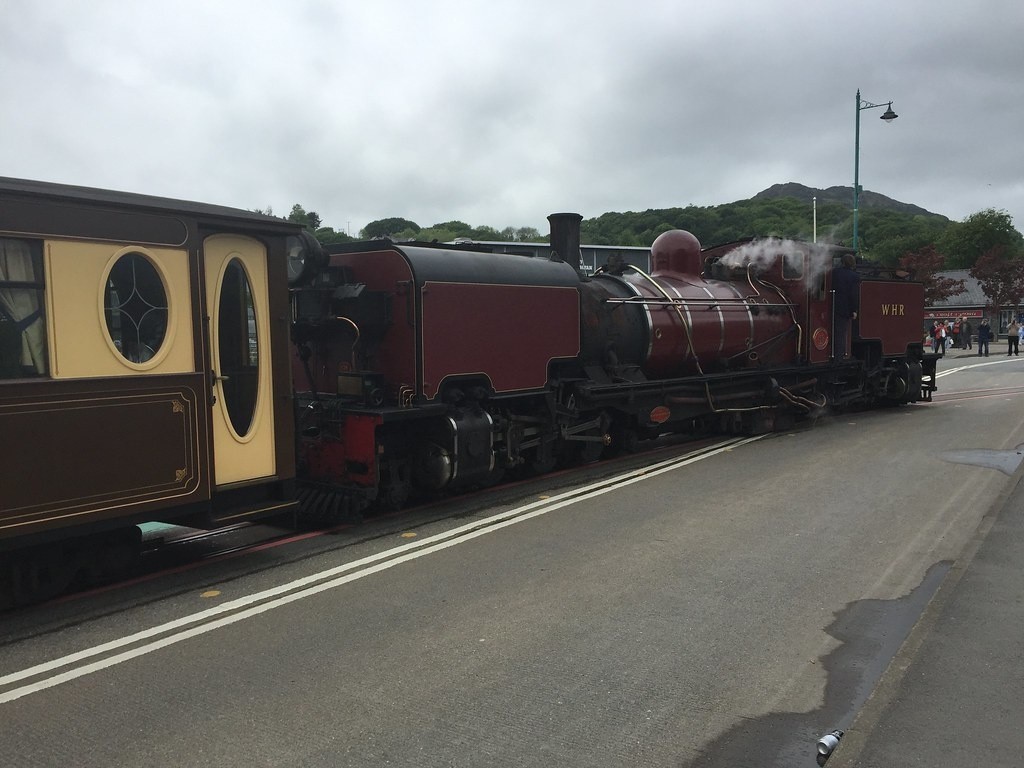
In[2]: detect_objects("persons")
[923,326,930,343]
[952,317,962,348]
[1006,318,1020,356]
[930,320,939,351]
[944,320,952,353]
[959,317,972,350]
[978,318,992,357]
[934,321,947,356]
[1018,322,1024,346]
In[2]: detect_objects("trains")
[0,172,948,610]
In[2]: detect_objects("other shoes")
[1014,352,1019,356]
[1007,354,1011,356]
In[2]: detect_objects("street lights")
[853,88,898,251]
